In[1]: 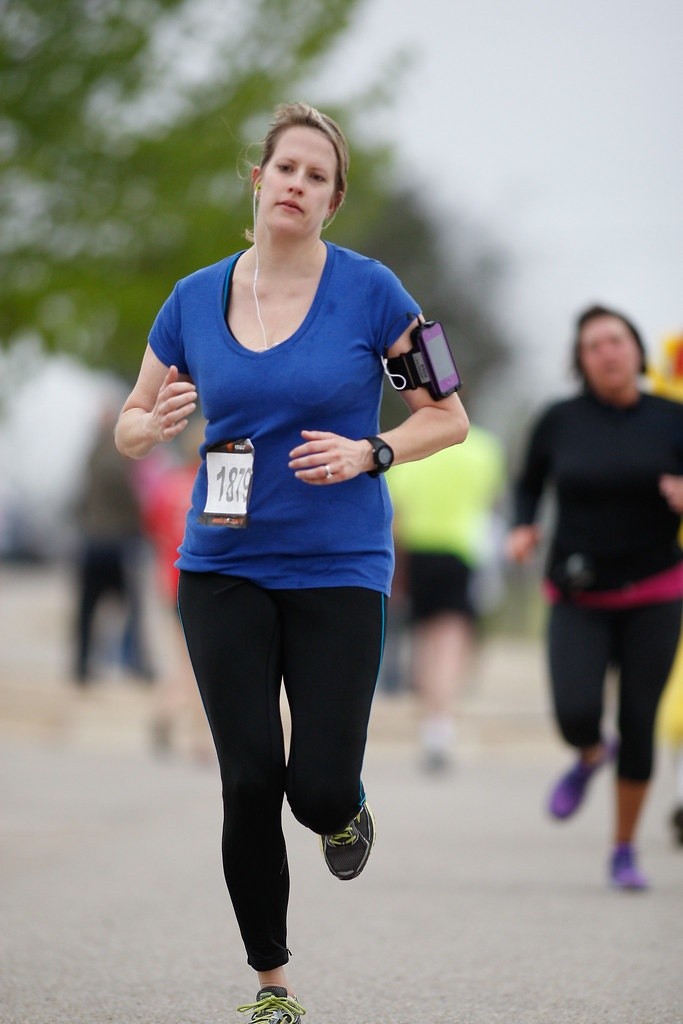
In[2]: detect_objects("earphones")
[254,182,261,195]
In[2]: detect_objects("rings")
[324,466,333,478]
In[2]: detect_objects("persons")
[149,456,201,746]
[386,428,505,767]
[504,305,683,887]
[55,402,157,691]
[116,104,469,1024]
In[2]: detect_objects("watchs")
[363,437,393,477]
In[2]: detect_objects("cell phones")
[417,320,460,397]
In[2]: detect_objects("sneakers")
[552,730,611,817]
[608,844,643,890]
[319,780,376,882]
[237,986,306,1024]
